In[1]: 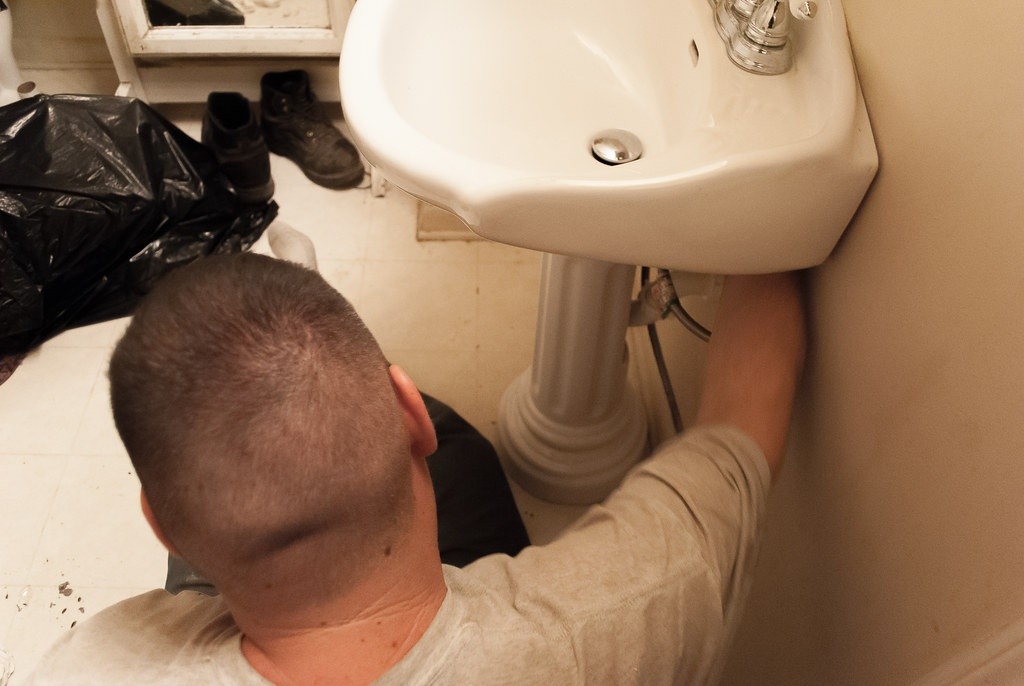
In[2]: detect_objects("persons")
[18,251,813,686]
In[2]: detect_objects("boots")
[260,68,363,190]
[201,92,276,200]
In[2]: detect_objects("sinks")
[337,0,881,273]
